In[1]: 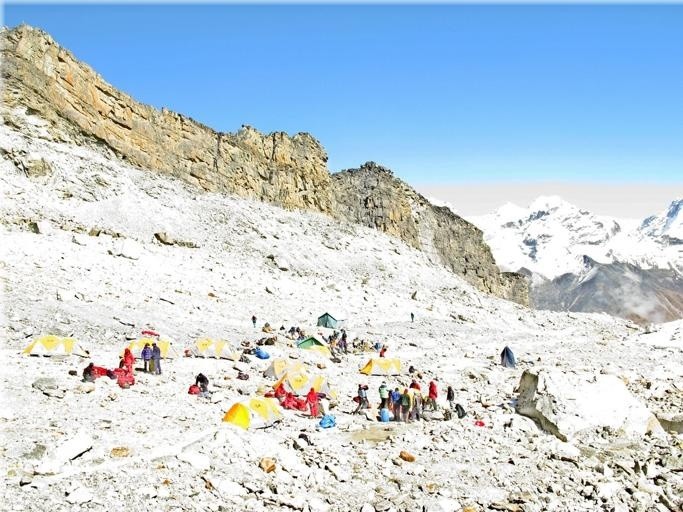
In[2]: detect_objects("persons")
[251,315,258,327]
[354,379,454,423]
[123,348,135,373]
[281,392,300,410]
[151,343,162,375]
[141,343,153,374]
[306,387,317,419]
[83,362,96,382]
[193,373,210,399]
[280,324,308,341]
[411,313,414,323]
[329,329,388,357]
[317,399,325,417]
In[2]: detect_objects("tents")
[119,337,179,360]
[306,345,334,361]
[293,337,325,350]
[264,357,326,397]
[359,358,401,376]
[222,397,285,432]
[194,338,235,361]
[21,336,88,358]
[316,312,347,329]
